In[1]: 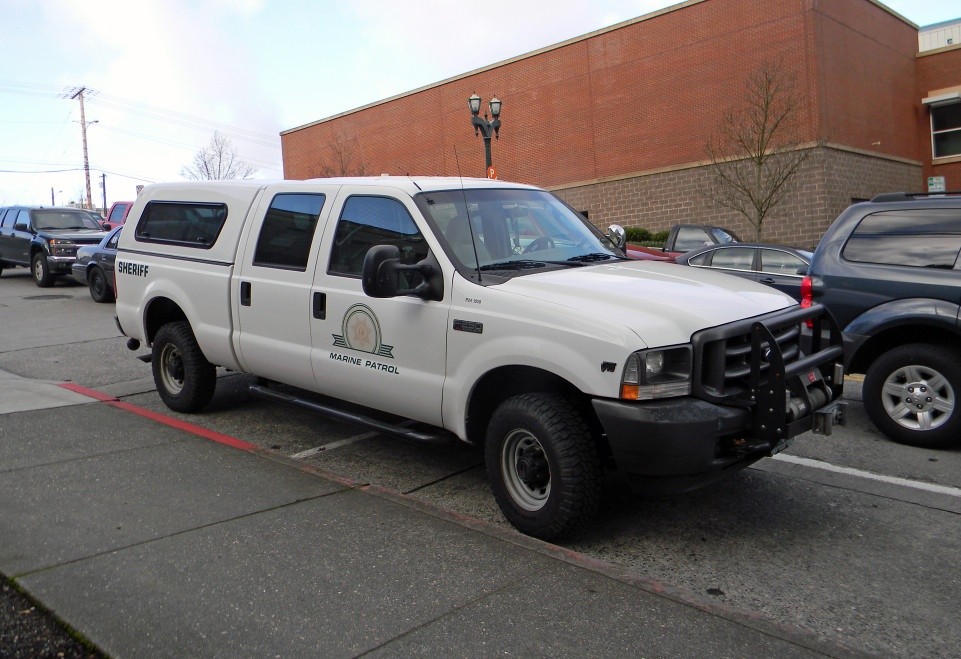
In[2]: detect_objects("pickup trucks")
[82,200,135,230]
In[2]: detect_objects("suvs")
[800,188,961,449]
[110,172,855,547]
[0,204,115,289]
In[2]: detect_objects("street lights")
[467,90,503,177]
[82,120,99,212]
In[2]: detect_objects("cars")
[444,200,815,304]
[70,224,125,304]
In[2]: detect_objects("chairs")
[447,218,489,266]
